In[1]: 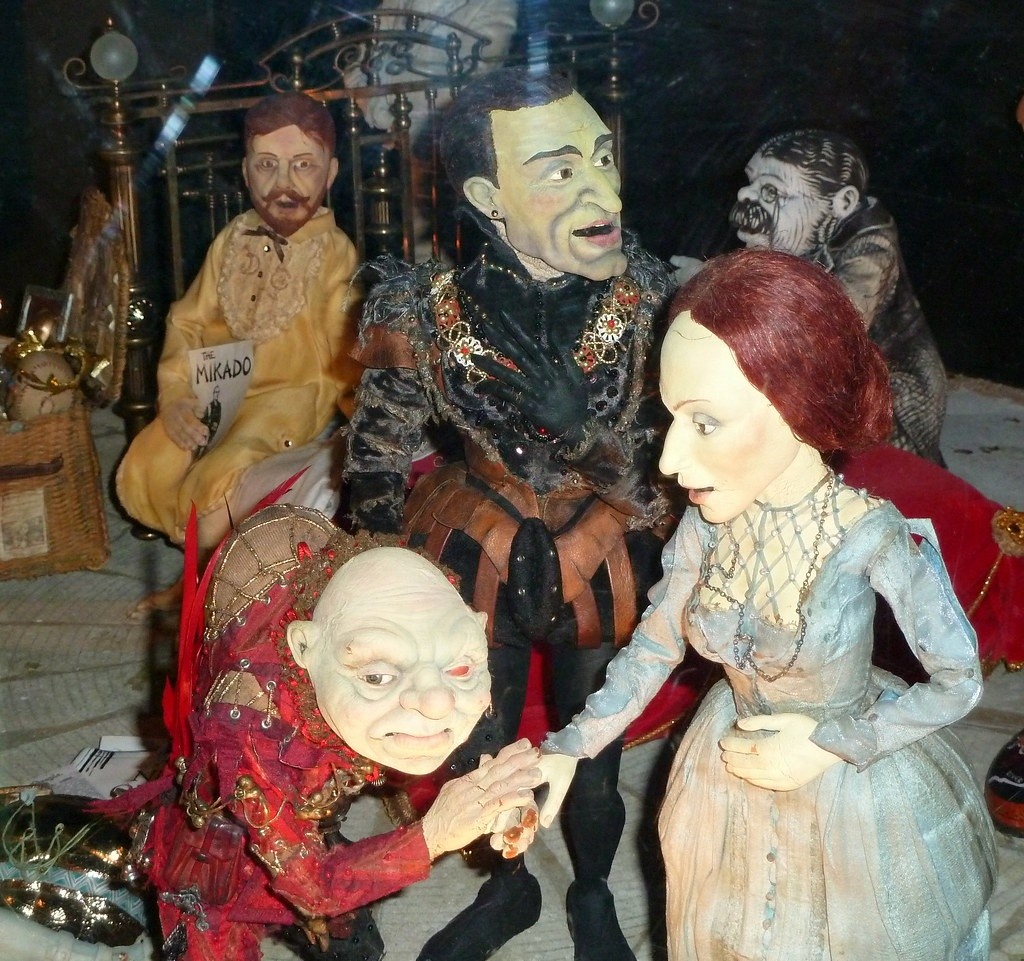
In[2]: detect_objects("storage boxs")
[0,217,129,582]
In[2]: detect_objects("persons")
[669,127,947,469]
[331,70,678,961]
[339,0,518,268]
[521,251,1002,961]
[120,502,542,961]
[115,93,361,547]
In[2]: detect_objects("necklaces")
[705,473,833,682]
